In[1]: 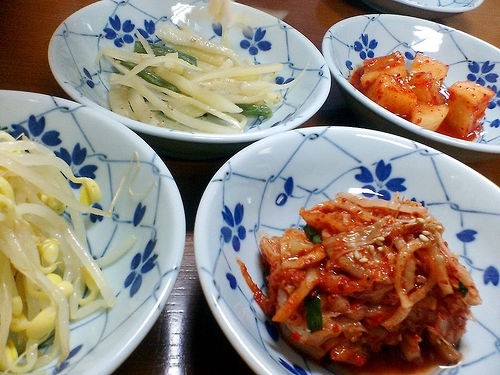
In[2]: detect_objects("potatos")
[98,22,309,133]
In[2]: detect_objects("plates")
[358,0,488,21]
[193,126,499,375]
[48,0,333,143]
[322,14,500,165]
[1,87,186,374]
